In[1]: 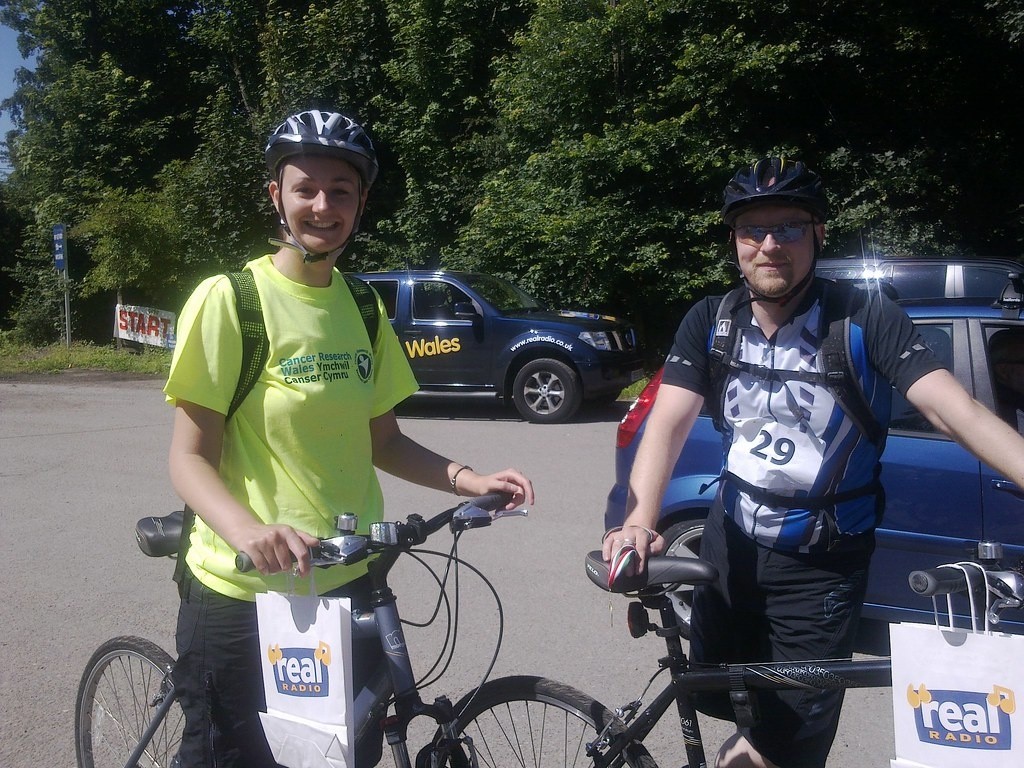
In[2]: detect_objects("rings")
[623,538,634,545]
[614,538,623,544]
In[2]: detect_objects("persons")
[163,110,536,767]
[602,154,1024,768]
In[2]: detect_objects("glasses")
[734,220,818,243]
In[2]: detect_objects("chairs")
[424,292,451,319]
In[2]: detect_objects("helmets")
[719,156,829,219]
[714,731,779,768]
[264,110,380,189]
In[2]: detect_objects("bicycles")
[423,475,1024,768]
[74,481,667,768]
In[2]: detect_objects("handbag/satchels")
[255,547,355,768]
[889,562,1024,767]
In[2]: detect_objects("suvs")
[583,251,1024,655]
[354,264,642,425]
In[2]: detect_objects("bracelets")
[449,464,473,498]
